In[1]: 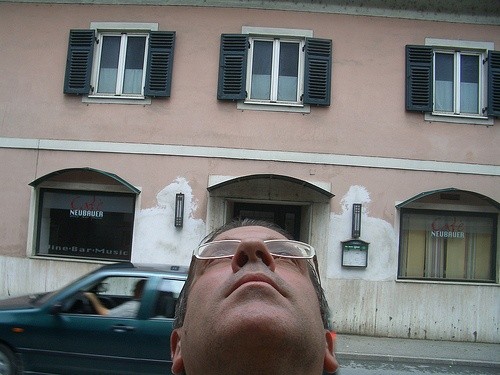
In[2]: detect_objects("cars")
[0,262,188,375]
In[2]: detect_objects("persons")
[84,279,146,318]
[168,220,340,375]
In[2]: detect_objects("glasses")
[189,239,322,284]
